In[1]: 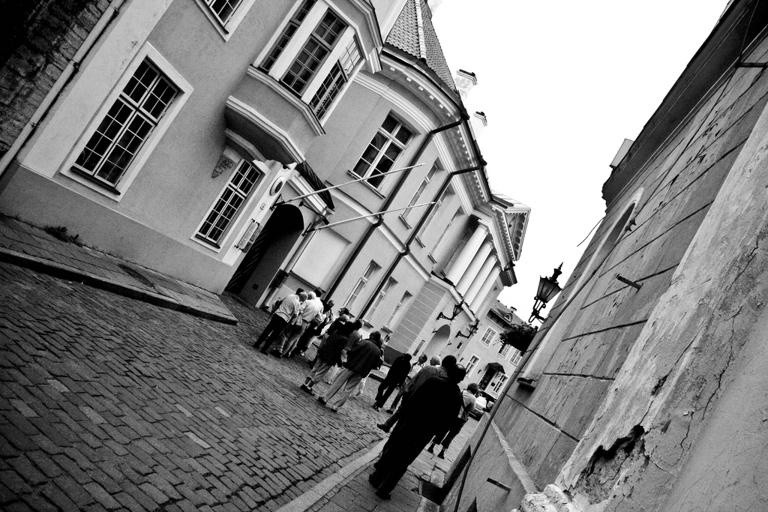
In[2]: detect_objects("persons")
[252,287,480,500]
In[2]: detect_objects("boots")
[438,449,444,459]
[428,443,435,453]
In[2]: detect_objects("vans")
[478,390,500,406]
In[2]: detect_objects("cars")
[468,397,488,421]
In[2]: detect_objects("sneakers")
[300,384,337,412]
[252,343,305,359]
[372,404,393,433]
